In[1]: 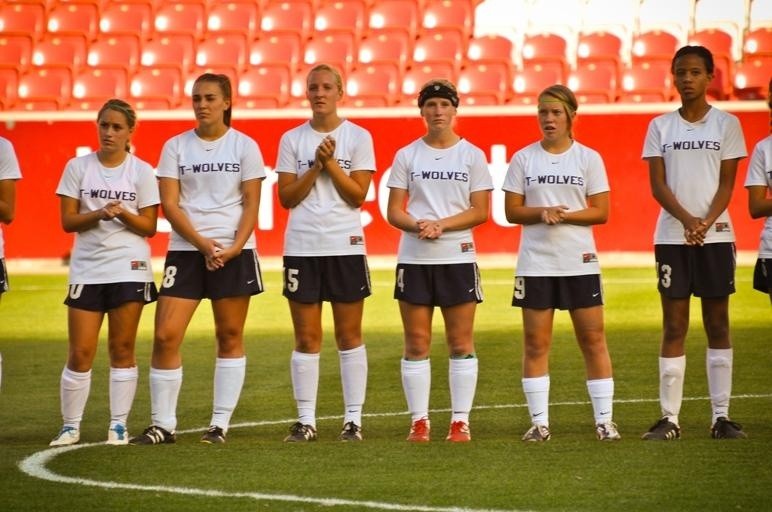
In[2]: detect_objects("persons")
[0,131,23,295]
[638,45,749,441]
[128,72,268,450]
[271,63,378,444]
[385,80,494,445]
[500,82,622,444]
[48,99,162,449]
[742,74,771,303]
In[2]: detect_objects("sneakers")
[597,421,621,440]
[445,421,472,443]
[49,426,81,446]
[644,419,684,442]
[285,424,317,442]
[522,425,549,442]
[106,425,129,447]
[337,420,363,442]
[407,419,432,442]
[711,417,747,439]
[128,427,174,446]
[199,425,228,445]
